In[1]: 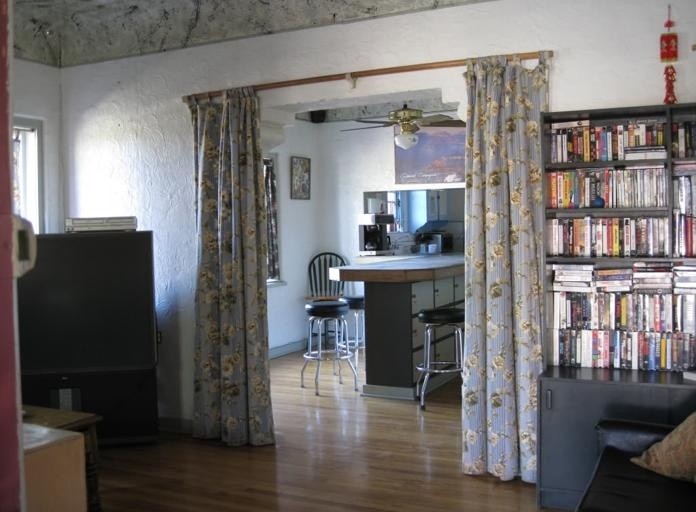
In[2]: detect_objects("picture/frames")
[328,253,468,401]
[22,400,104,511]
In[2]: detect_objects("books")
[415,230,454,254]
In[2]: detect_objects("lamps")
[303,251,347,351]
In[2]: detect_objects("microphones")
[414,307,466,410]
[299,298,361,397]
[332,294,371,393]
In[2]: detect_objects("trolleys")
[339,101,469,133]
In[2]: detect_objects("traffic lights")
[630,411,696,481]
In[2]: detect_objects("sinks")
[574,416,696,509]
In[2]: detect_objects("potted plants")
[290,156,312,200]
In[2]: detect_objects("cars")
[420,244,428,253]
[429,245,436,254]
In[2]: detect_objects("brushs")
[357,213,394,256]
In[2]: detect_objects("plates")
[21,421,88,512]
[411,190,447,236]
[537,380,696,510]
[541,102,696,364]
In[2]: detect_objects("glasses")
[394,129,422,150]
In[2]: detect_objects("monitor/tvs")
[547,122,696,372]
